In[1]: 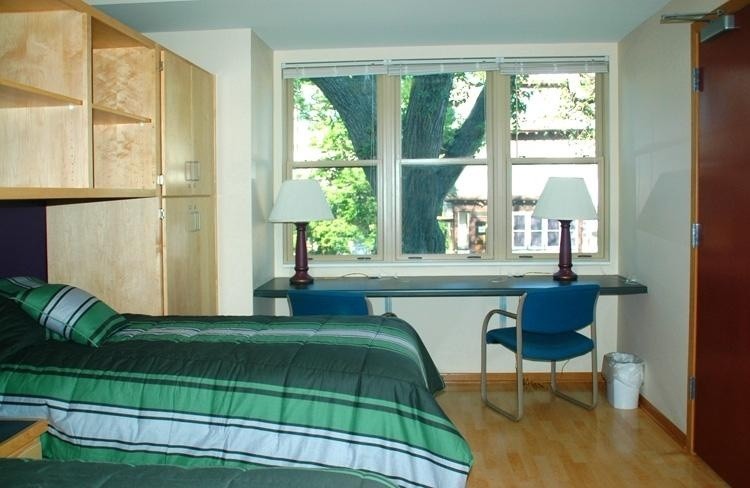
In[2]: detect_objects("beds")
[0,275,472,488]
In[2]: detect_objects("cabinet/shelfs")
[46,44,220,319]
[0,0,162,199]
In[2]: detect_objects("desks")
[254,274,647,330]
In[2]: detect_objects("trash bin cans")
[604,353,646,410]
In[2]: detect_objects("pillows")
[0,275,126,361]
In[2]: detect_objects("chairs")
[479,284,601,423]
[285,289,373,316]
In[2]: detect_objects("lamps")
[268,179,336,284]
[531,176,600,284]
